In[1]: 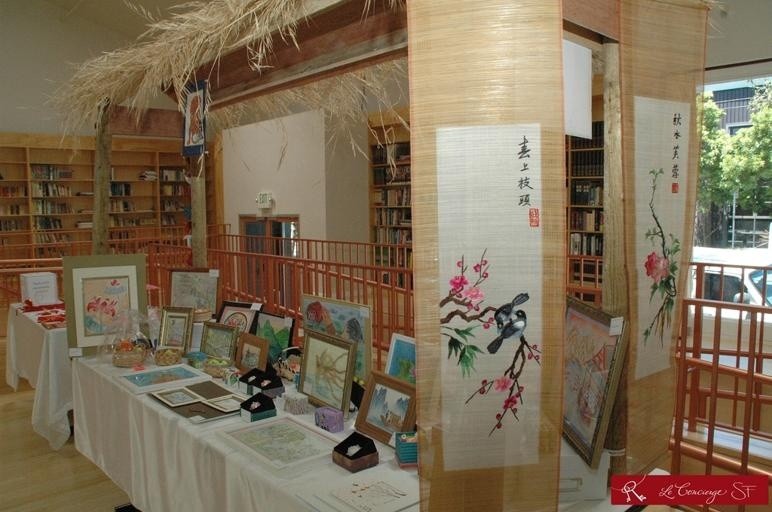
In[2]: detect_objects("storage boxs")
[19,272,58,305]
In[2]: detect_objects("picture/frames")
[218,413,342,470]
[561,293,631,469]
[331,470,420,512]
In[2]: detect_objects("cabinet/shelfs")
[0,130,214,337]
[367,74,604,309]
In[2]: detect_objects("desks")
[71,319,420,511]
[4,298,165,451]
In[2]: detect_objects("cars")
[683,246,772,357]
